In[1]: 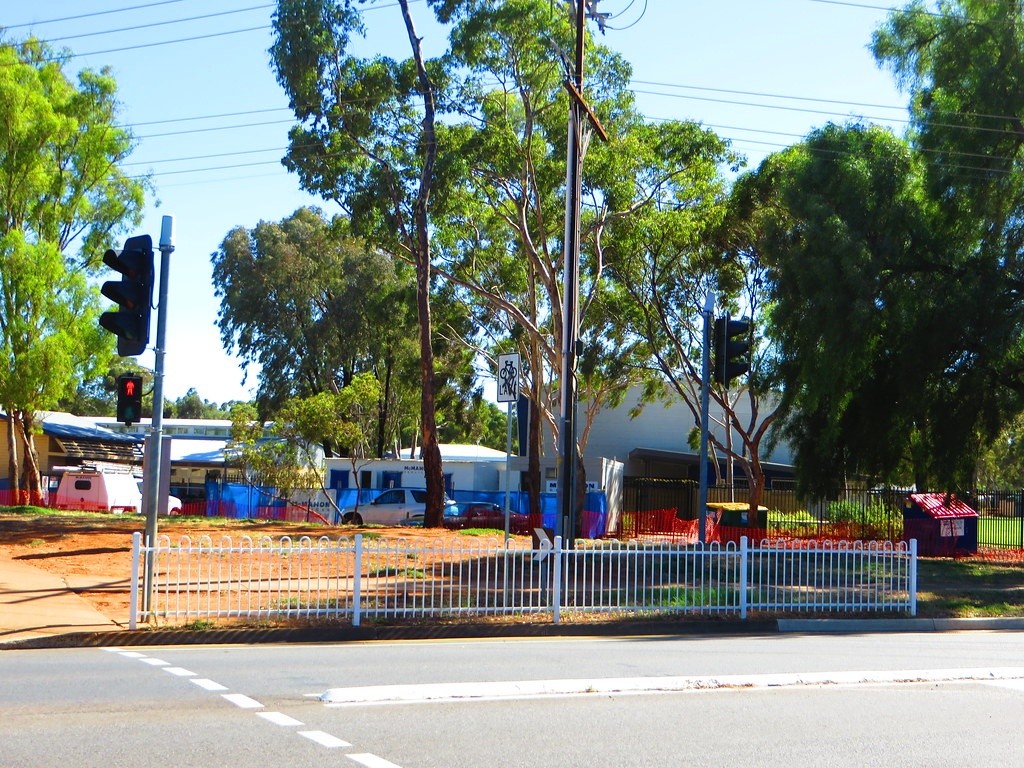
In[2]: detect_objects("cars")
[399,500,531,536]
[338,486,459,528]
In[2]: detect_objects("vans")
[168,485,206,504]
[55,462,183,517]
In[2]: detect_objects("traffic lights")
[118,371,142,427]
[714,311,749,392]
[98,234,154,357]
